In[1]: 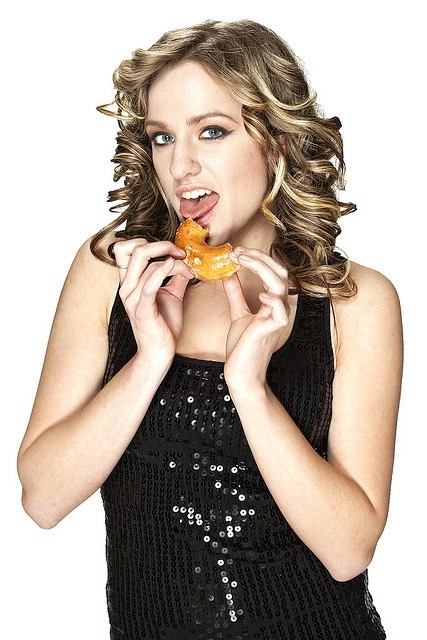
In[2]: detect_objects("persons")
[16,19,403,640]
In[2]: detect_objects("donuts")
[175,218,242,282]
